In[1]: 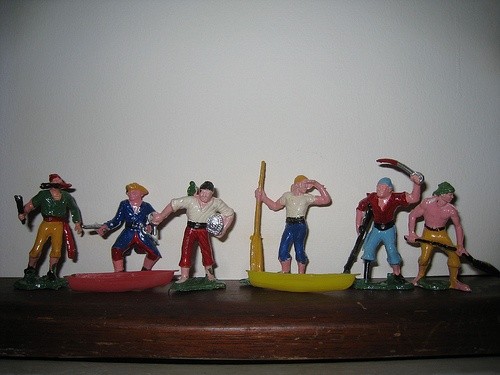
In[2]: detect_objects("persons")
[408,181,471,291]
[152,181,234,283]
[356,171,425,284]
[254,175,331,274]
[97,182,162,272]
[18,174,82,282]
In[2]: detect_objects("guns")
[342,205,376,275]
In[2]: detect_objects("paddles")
[249,161,271,273]
[405,234,496,279]
